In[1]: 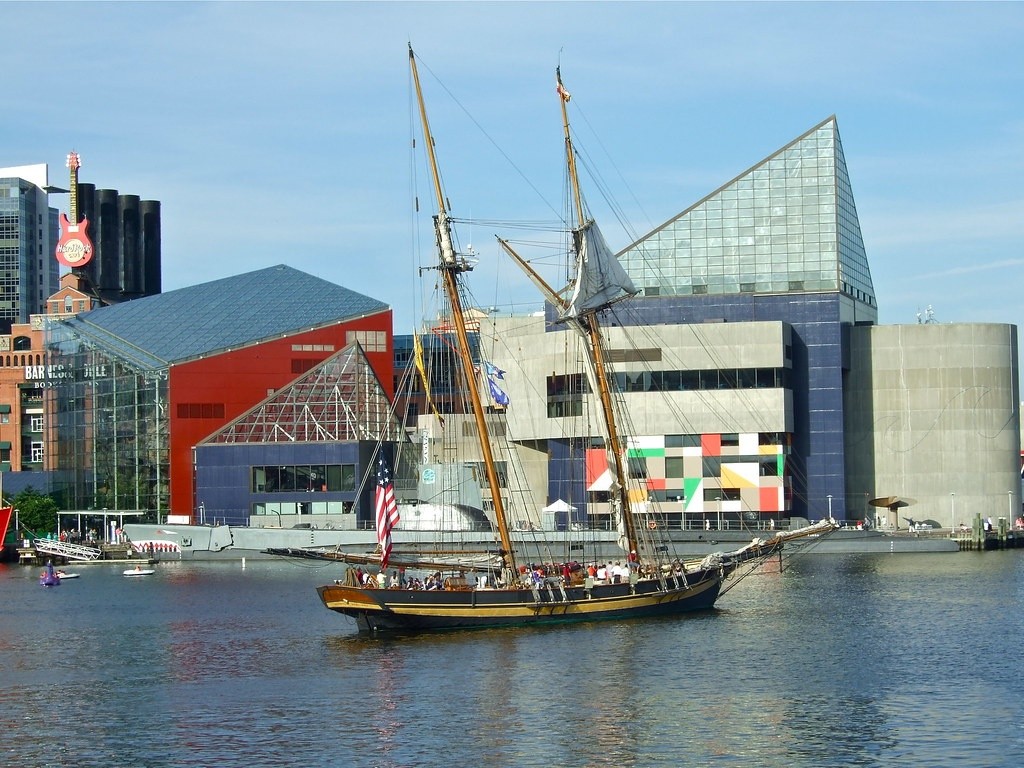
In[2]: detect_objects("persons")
[702,512,1023,542]
[45,528,154,572]
[356,549,640,591]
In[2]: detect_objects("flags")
[485,361,506,380]
[487,376,510,406]
[375,445,401,570]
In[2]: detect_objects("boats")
[40,570,80,580]
[40,559,61,586]
[123,569,155,575]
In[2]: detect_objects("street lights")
[951,493,955,533]
[826,494,832,519]
[715,497,722,530]
[1008,490,1013,531]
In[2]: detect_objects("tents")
[542,498,579,532]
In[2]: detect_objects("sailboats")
[259,37,844,640]
